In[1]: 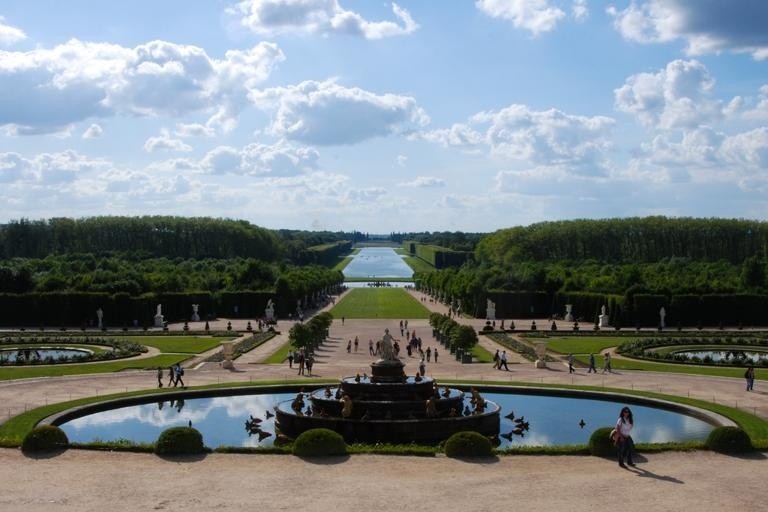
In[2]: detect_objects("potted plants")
[429,311,478,363]
[288,312,334,363]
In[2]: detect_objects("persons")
[379,327,401,359]
[298,310,305,324]
[297,350,305,376]
[169,399,174,408]
[305,352,314,376]
[156,399,164,409]
[613,403,636,470]
[744,361,757,392]
[156,365,163,388]
[286,348,294,368]
[368,339,375,355]
[602,352,609,374]
[175,398,184,413]
[341,314,345,326]
[417,356,426,375]
[166,365,176,387]
[400,318,439,362]
[568,351,575,373]
[346,339,352,353]
[374,341,381,355]
[354,335,360,352]
[393,341,400,356]
[493,348,501,369]
[457,303,462,316]
[607,350,612,371]
[173,362,184,389]
[498,350,509,371]
[587,352,597,374]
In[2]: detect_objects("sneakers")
[620,462,636,468]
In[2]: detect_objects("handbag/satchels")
[610,429,620,443]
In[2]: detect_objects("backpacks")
[180,368,184,376]
